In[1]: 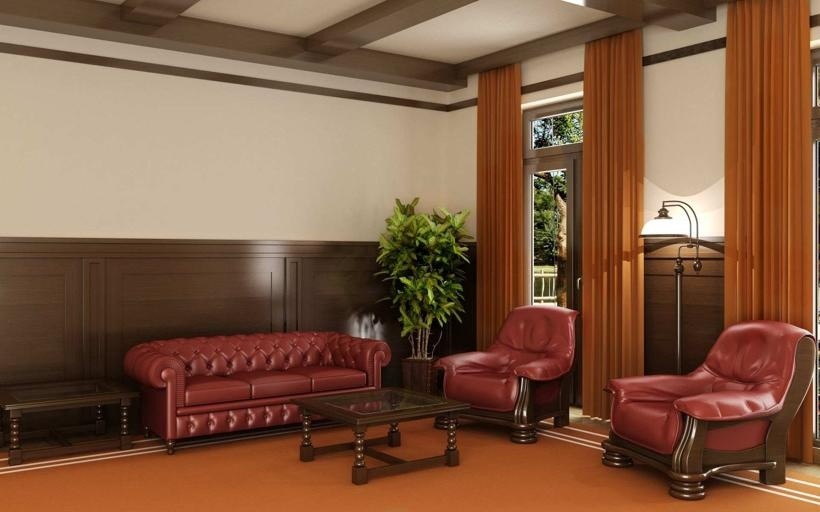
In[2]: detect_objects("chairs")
[433,302,581,444]
[602,318,819,504]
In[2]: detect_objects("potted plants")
[372,196,477,396]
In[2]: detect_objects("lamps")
[637,199,704,377]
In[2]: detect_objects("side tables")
[1,378,141,465]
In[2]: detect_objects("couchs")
[121,329,391,455]
